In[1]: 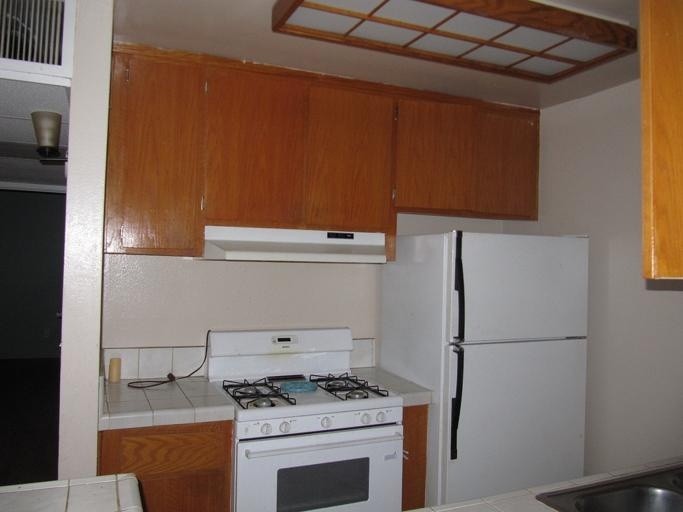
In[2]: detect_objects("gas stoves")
[209,326,403,441]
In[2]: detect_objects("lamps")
[31,111,68,165]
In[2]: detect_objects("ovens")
[230,406,403,512]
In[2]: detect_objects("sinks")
[668,463,683,492]
[536,484,683,512]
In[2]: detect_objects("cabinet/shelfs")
[395,86,540,220]
[97,420,233,512]
[402,404,428,511]
[203,53,398,261]
[104,42,205,257]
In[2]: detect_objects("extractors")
[195,225,387,264]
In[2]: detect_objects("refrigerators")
[376,231,589,508]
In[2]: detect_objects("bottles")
[108,358,121,383]
